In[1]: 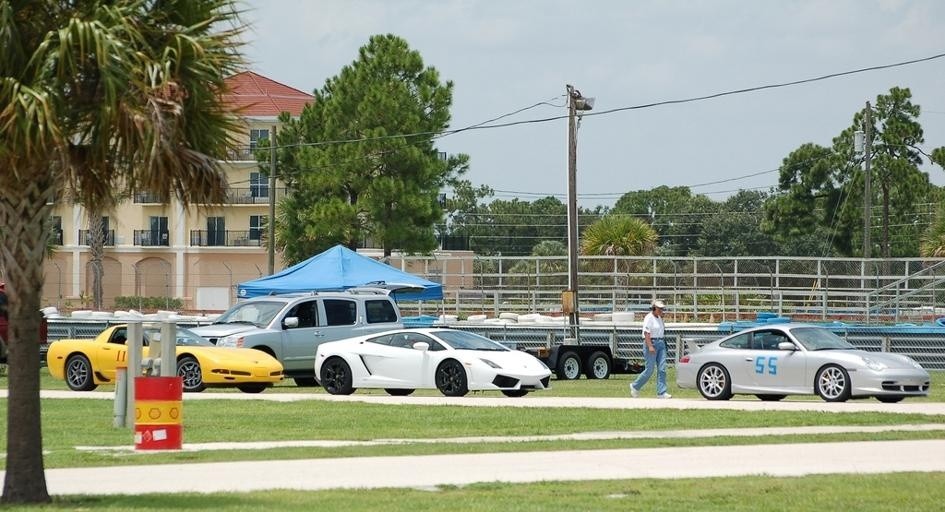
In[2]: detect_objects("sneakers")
[657,393,672,399]
[629,384,639,397]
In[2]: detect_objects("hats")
[652,300,665,308]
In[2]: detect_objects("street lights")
[564,81,595,340]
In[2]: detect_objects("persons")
[629,299,673,397]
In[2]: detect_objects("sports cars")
[312,326,553,398]
[44,323,285,394]
[671,321,930,404]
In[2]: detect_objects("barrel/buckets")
[135,374,183,450]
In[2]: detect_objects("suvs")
[184,286,403,386]
